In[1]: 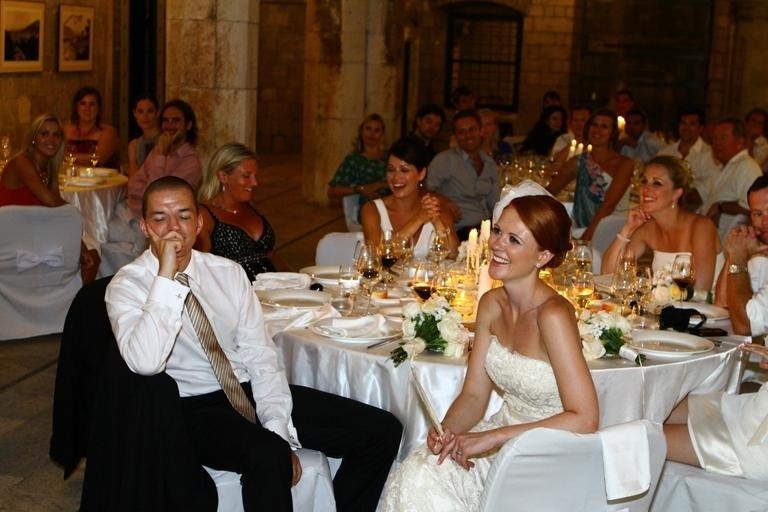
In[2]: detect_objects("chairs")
[337,118,767,264]
[1,116,181,344]
[75,228,767,511]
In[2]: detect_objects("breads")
[373,290,388,298]
[590,294,603,300]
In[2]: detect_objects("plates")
[256,288,333,311]
[70,178,104,187]
[628,327,714,356]
[300,264,359,285]
[313,318,402,344]
[675,301,732,324]
[92,168,115,177]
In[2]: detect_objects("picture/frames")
[0,3,45,74]
[57,3,92,73]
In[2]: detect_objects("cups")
[0,137,100,180]
[496,154,550,191]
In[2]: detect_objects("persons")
[104,177,403,512]
[327,85,768,335]
[378,196,599,512]
[661,343,768,479]
[1,86,274,287]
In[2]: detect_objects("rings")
[456,449,463,456]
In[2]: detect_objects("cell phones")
[690,328,728,336]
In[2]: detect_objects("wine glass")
[339,229,700,342]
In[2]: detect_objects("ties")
[175,273,256,425]
